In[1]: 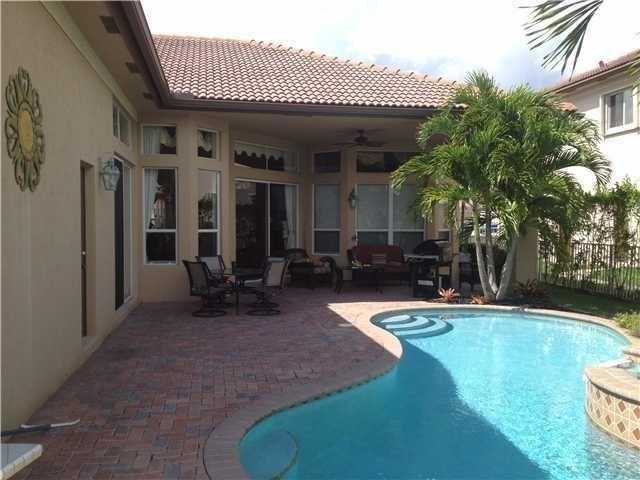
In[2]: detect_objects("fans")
[332,129,389,149]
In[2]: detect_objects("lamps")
[348,187,360,209]
[98,159,121,192]
